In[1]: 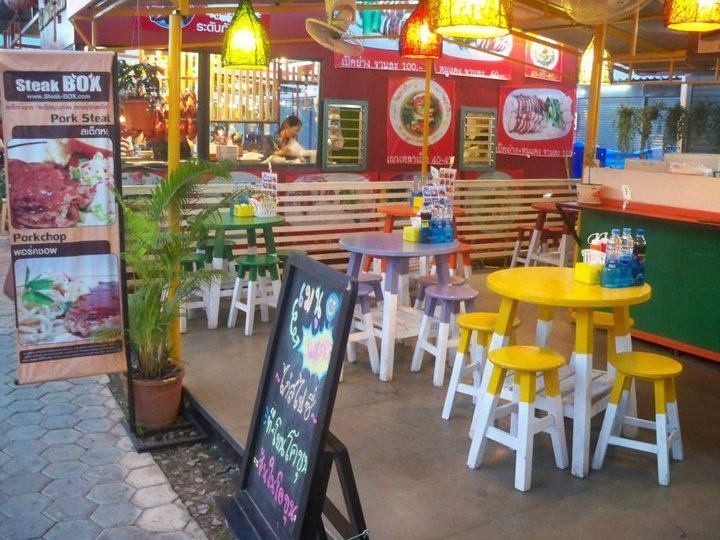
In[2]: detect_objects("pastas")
[16,271,92,344]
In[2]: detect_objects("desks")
[339,231,461,380]
[184,211,288,331]
[526,200,583,268]
[371,203,465,281]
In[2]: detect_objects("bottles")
[229,179,259,214]
[419,196,456,243]
[356,0,418,38]
[586,224,648,288]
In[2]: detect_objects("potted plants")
[100,154,261,433]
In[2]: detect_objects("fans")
[306,1,366,62]
[557,0,653,26]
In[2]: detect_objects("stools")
[175,238,697,496]
[359,221,604,275]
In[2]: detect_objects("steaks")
[6,157,94,229]
[67,281,120,336]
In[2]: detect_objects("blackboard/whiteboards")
[235,251,359,540]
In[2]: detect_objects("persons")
[261,116,308,164]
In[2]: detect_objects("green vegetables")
[545,97,563,131]
[402,91,443,138]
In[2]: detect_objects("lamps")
[216,0,272,70]
[661,0,720,34]
[395,0,512,61]
